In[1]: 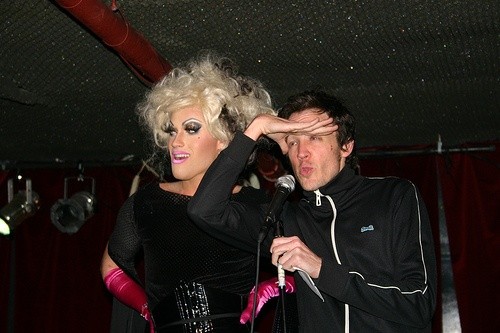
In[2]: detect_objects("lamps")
[49,162,98,233]
[0,174,40,235]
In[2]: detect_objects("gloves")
[103,268,155,333]
[239,274,296,324]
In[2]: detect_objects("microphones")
[257,175,295,241]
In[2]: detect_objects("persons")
[190,92,436,333]
[100,51,295,333]
